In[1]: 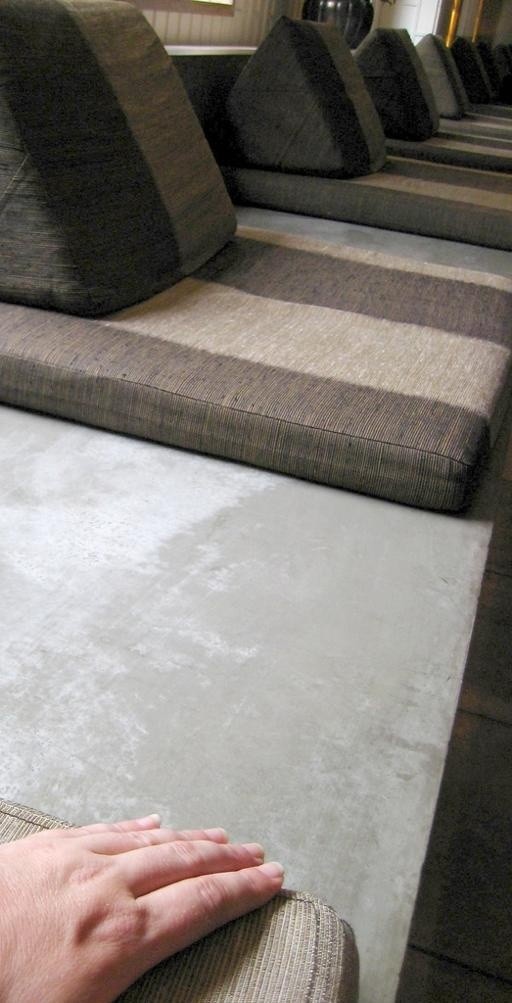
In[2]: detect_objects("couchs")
[0,0,512,519]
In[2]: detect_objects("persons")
[0,813,285,1003]
